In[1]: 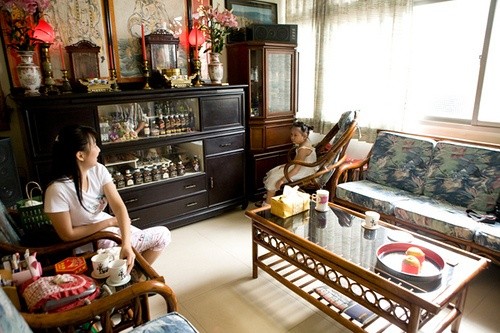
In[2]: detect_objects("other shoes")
[255,200,265,207]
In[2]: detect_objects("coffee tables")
[245,202,492,333]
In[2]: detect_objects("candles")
[194,22,198,59]
[141,24,146,60]
[60,45,65,70]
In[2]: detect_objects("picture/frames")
[0,0,213,97]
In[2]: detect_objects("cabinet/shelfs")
[227,24,298,205]
[16,81,249,230]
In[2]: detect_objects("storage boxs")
[55,257,88,275]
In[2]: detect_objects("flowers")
[0,0,51,51]
[198,4,238,55]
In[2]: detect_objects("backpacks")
[486,192,500,223]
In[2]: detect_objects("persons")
[255,121,317,207]
[102,103,147,136]
[42,124,172,296]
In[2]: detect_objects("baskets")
[16,181,52,233]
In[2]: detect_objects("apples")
[401,246,425,275]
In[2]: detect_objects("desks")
[21,246,149,333]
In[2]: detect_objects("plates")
[106,274,131,286]
[91,269,110,279]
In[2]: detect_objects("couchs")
[332,129,500,264]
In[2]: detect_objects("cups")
[90,252,115,276]
[364,210,380,227]
[107,259,129,283]
[311,189,329,211]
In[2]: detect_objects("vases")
[16,51,42,96]
[208,53,223,85]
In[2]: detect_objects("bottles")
[177,160,185,176]
[250,62,291,118]
[169,161,177,178]
[99,104,194,143]
[160,163,169,179]
[110,174,116,188]
[125,169,134,187]
[151,165,161,181]
[134,168,143,184]
[116,172,125,189]
[192,156,199,172]
[143,166,152,182]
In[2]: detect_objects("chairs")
[0,203,199,333]
[275,110,359,196]
